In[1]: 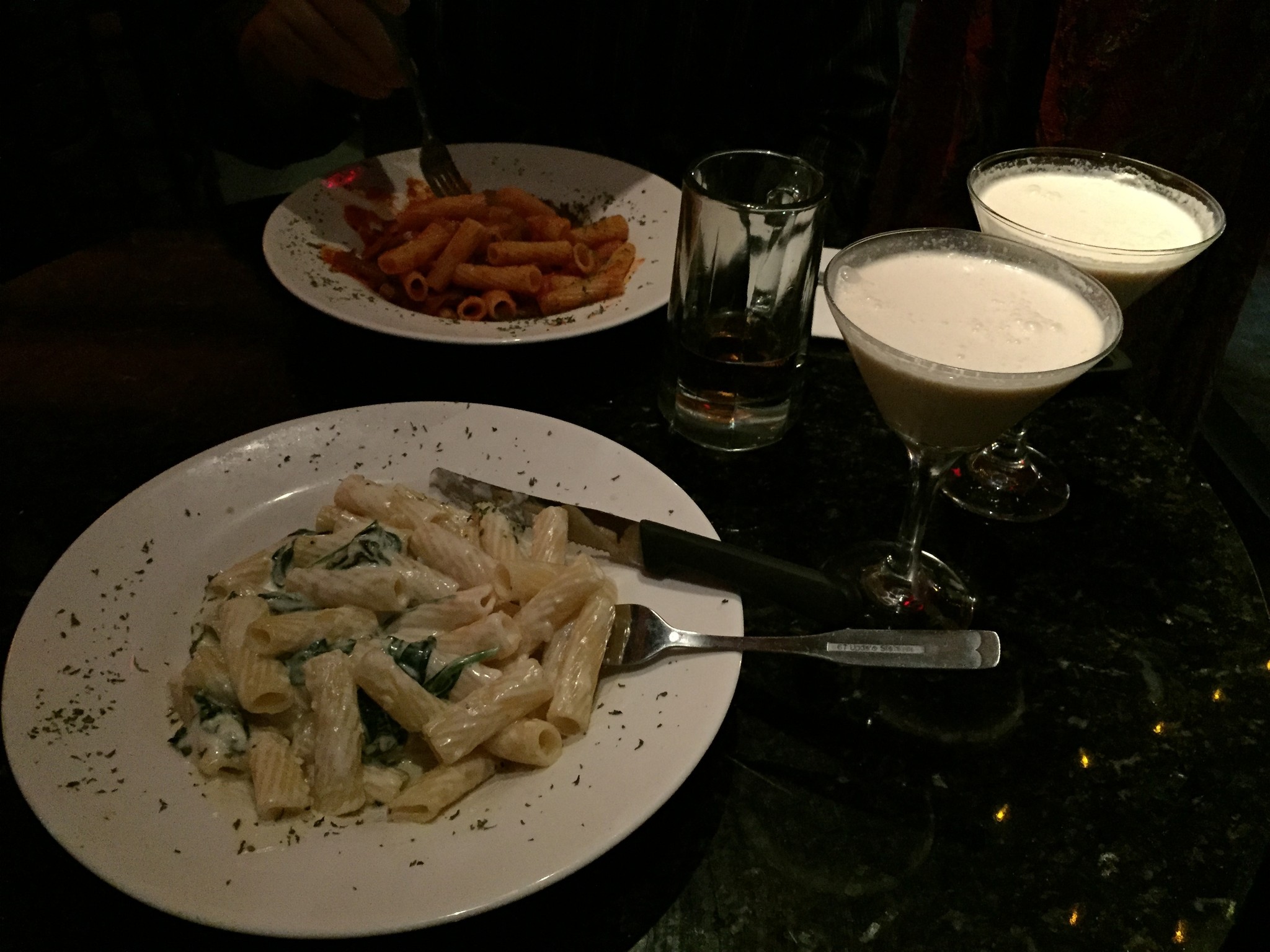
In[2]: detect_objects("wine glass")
[933,144,1227,526]
[823,226,1126,629]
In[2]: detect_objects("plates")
[0,400,746,938]
[263,143,683,343]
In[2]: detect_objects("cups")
[662,149,826,457]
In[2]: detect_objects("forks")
[600,603,1002,672]
[371,0,471,199]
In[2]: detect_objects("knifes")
[427,467,851,614]
